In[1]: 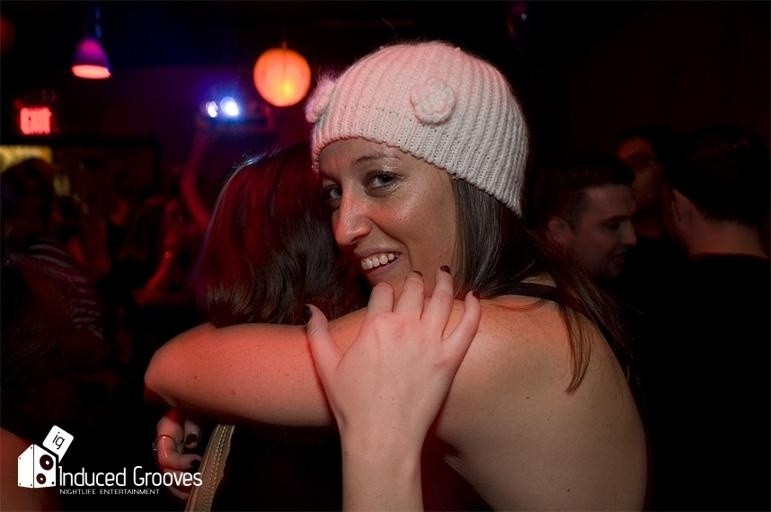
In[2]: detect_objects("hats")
[301,38,533,222]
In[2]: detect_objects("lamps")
[254,48,313,108]
[69,34,112,80]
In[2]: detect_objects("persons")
[0,102,283,511]
[522,142,638,284]
[601,127,681,278]
[142,40,651,511]
[181,141,483,511]
[613,123,771,512]
